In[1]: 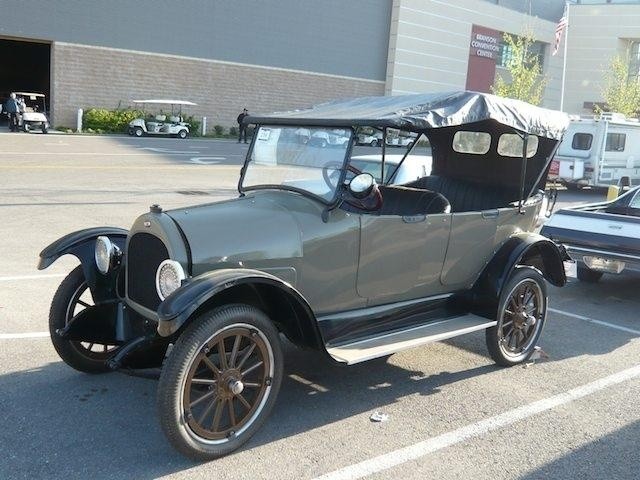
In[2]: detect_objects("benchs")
[146,115,180,127]
[25,107,34,112]
[327,176,518,215]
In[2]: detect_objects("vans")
[547,110,640,197]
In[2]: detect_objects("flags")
[549,1,569,56]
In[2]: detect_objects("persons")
[237,107,252,146]
[18,97,26,115]
[6,92,21,132]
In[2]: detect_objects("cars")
[36,88,570,464]
[539,183,640,282]
